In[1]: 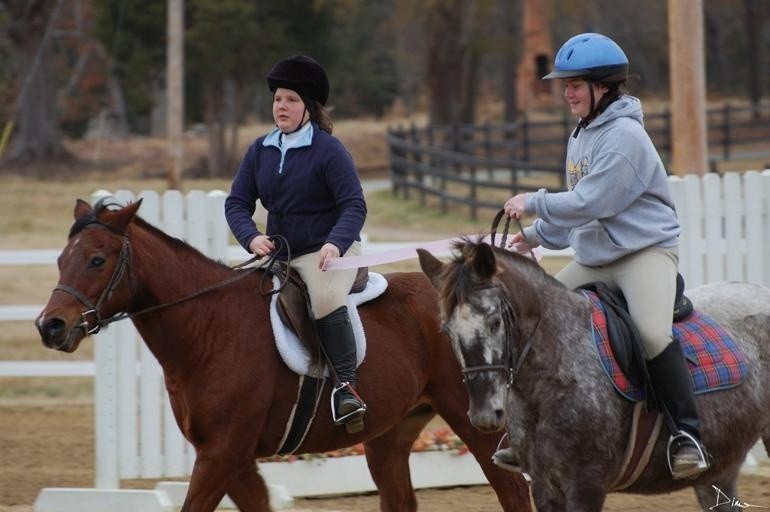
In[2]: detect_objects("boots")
[646,339,711,481]
[315,304,367,435]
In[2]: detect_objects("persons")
[502,31,720,483]
[224,51,367,436]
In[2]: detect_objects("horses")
[34,194,534,512]
[415,227,770,512]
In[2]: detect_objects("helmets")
[265,54,330,107]
[541,32,629,84]
